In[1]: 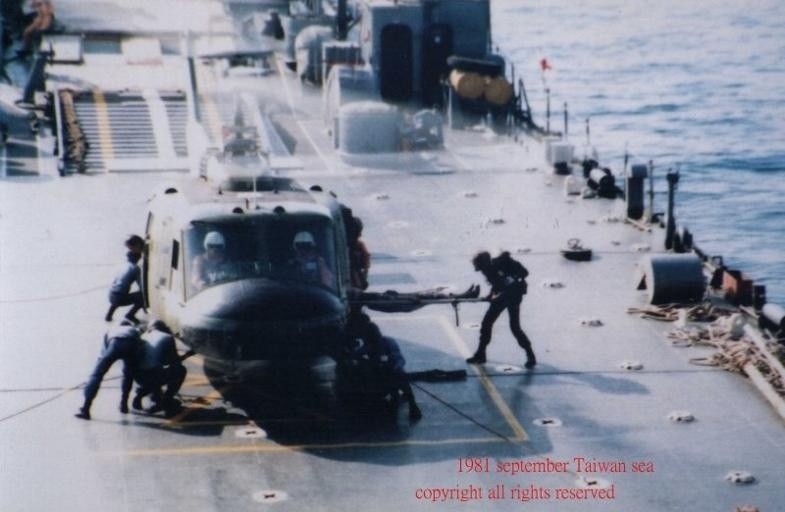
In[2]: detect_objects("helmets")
[293,231,315,248]
[203,231,226,250]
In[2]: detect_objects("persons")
[471,250,535,368]
[350,216,372,291]
[74,320,200,419]
[190,232,236,289]
[411,102,443,146]
[285,233,337,293]
[349,282,481,311]
[334,317,425,438]
[13,0,54,58]
[102,250,144,325]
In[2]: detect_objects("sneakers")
[133,392,182,418]
[75,406,92,419]
[104,309,115,321]
[524,352,536,368]
[125,309,142,324]
[465,351,487,363]
[119,395,129,412]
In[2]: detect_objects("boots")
[466,283,479,298]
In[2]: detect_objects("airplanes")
[135,41,373,381]
